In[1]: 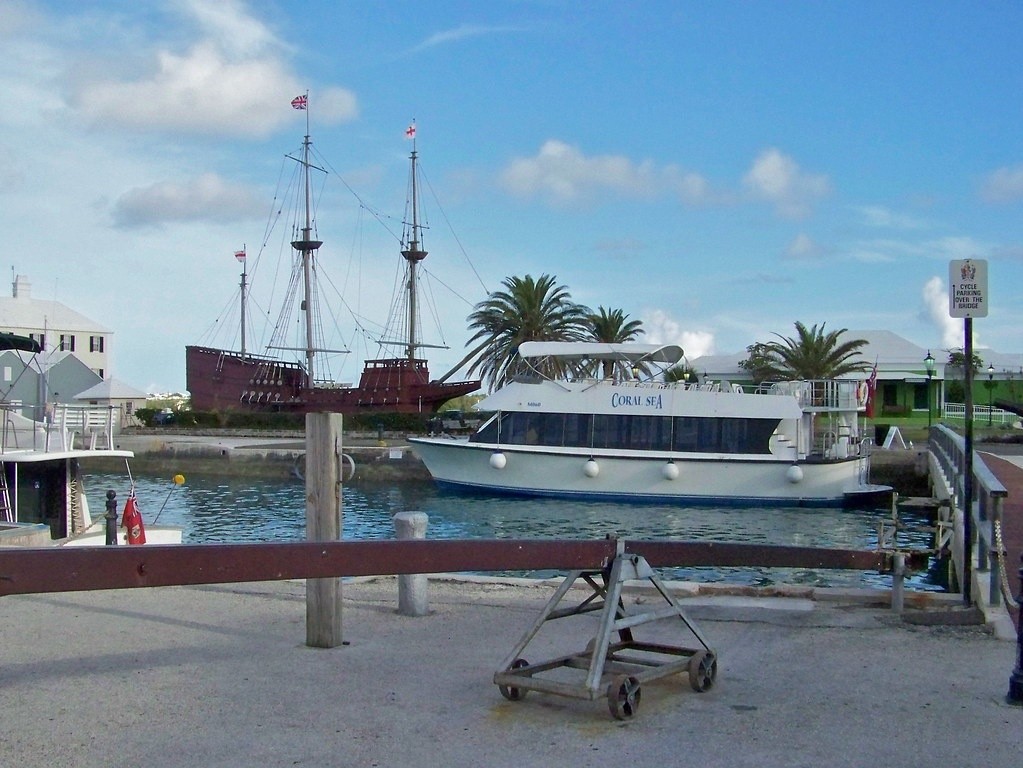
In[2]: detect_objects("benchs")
[36,404,117,453]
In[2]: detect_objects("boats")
[404,341,894,511]
[0,403,184,546]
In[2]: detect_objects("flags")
[232,251,247,263]
[290,95,307,110]
[867,365,877,390]
[405,123,416,139]
[120,485,147,545]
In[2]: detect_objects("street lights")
[923,348,935,430]
[987,361,996,428]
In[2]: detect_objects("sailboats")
[185,88,482,417]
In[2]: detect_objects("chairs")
[582,378,812,405]
